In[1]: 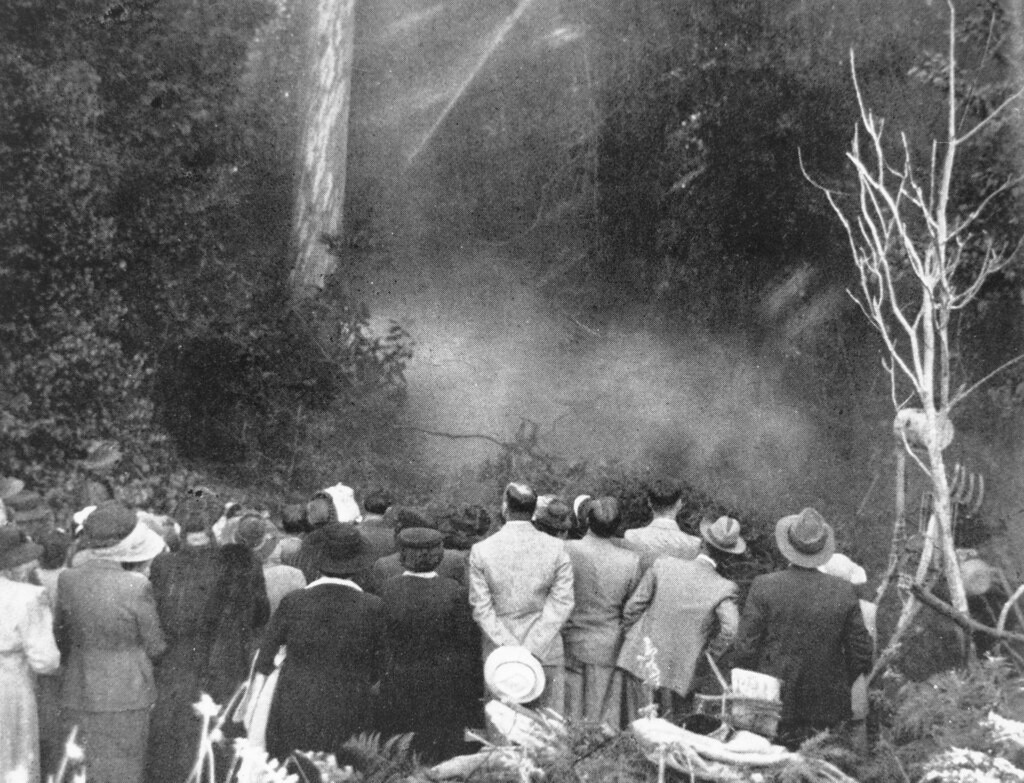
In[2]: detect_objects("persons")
[1,473,877,783]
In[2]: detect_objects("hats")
[174,496,225,533]
[821,554,869,585]
[699,514,747,556]
[0,526,46,569]
[398,527,442,549]
[228,514,279,558]
[484,647,546,704]
[302,522,378,573]
[71,504,167,561]
[541,499,572,529]
[776,508,834,568]
